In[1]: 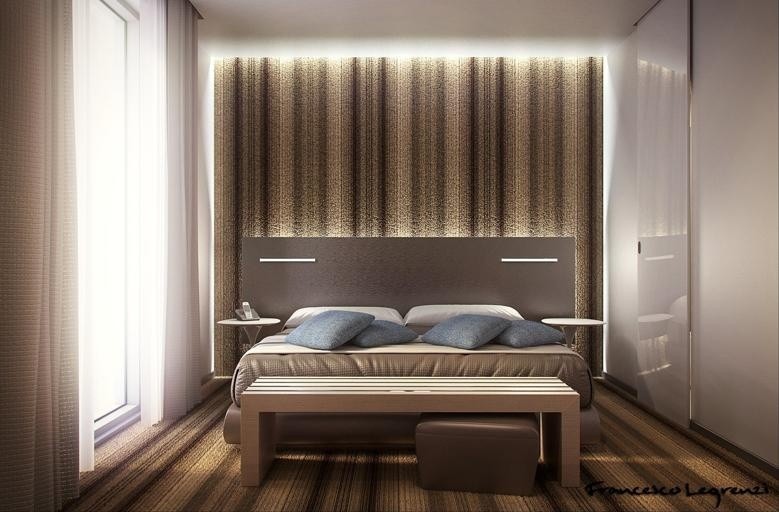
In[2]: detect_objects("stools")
[415,413,541,497]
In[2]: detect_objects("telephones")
[235,301,260,321]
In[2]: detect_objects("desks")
[540,318,606,348]
[216,318,281,347]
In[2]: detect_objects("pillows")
[282,302,565,348]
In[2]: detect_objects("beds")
[225,237,603,448]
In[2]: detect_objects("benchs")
[240,375,579,489]
